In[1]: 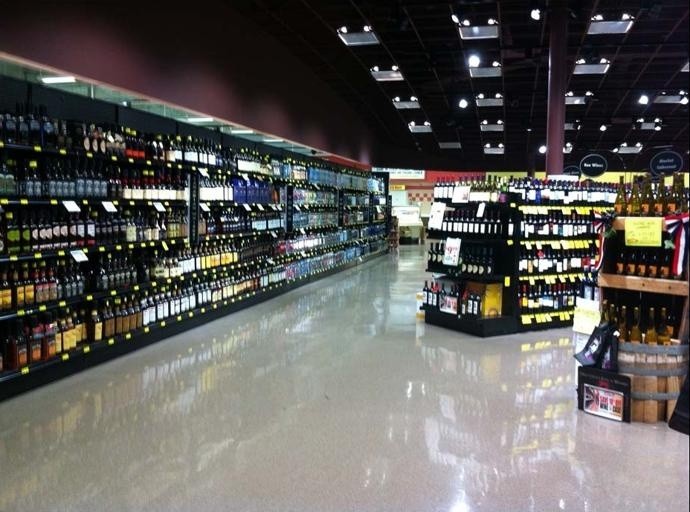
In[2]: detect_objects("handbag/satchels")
[574,320,618,371]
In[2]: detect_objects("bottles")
[0,103,390,378]
[422,169,690,345]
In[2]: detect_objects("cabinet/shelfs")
[419,198,690,346]
[0,76,398,404]
